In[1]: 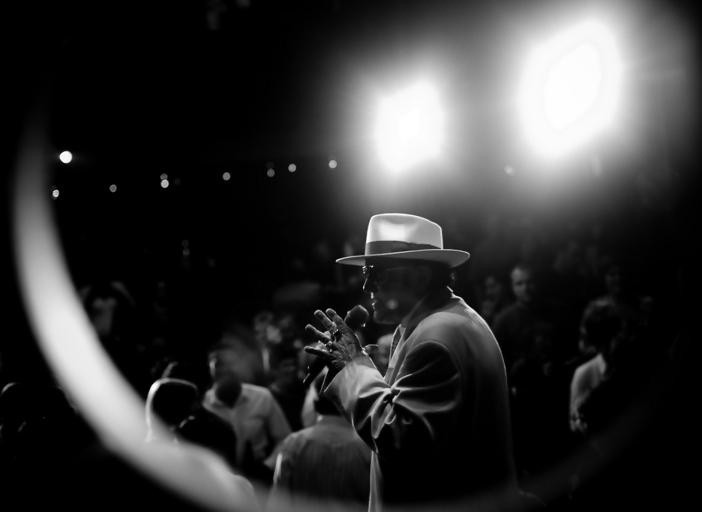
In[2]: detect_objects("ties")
[389,327,402,358]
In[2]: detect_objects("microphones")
[303,305,371,385]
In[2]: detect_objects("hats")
[336,212,471,270]
